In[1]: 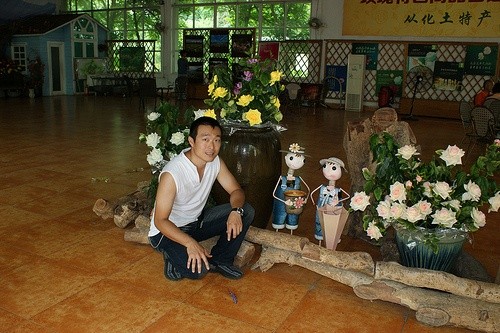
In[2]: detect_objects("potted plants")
[187,67,211,100]
[27,56,44,96]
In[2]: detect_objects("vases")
[211,127,283,229]
[29,89,35,98]
[394,227,465,273]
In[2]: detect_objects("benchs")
[155,87,173,100]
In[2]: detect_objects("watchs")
[231,208,244,215]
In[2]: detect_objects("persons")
[310,156,352,246]
[476,79,500,142]
[272,143,310,235]
[177,50,189,97]
[148,116,254,280]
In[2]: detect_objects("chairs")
[459,98,500,165]
[281,80,329,117]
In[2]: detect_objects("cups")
[284,190,307,215]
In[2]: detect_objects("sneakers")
[208,259,244,280]
[161,248,183,280]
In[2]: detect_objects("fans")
[307,17,321,32]
[154,22,168,35]
[401,65,434,121]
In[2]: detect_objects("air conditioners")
[345,55,367,112]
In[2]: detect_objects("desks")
[378,86,402,108]
[90,76,156,99]
[0,87,28,98]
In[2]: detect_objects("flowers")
[142,101,217,212]
[350,130,500,245]
[203,56,288,134]
